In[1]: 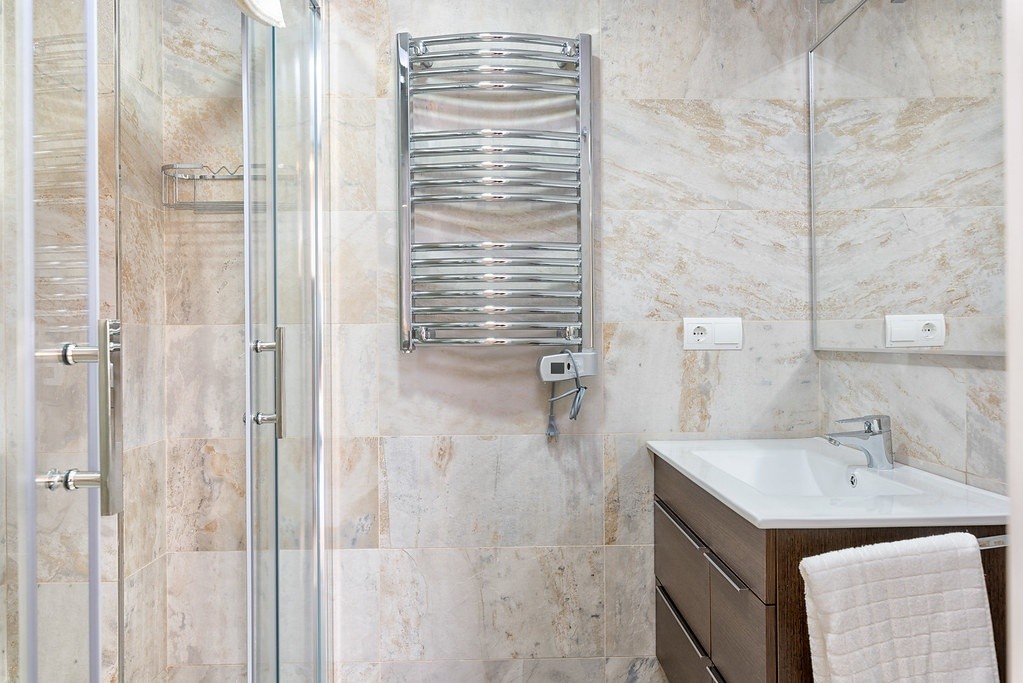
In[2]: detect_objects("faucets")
[824,415,893,470]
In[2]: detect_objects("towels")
[798,532,1001,683]
[234,0,286,28]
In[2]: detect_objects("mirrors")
[807,1,1005,359]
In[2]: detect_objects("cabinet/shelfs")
[644,436,1009,683]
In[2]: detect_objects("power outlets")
[886,313,946,348]
[682,317,743,350]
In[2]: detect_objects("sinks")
[690,449,925,497]
